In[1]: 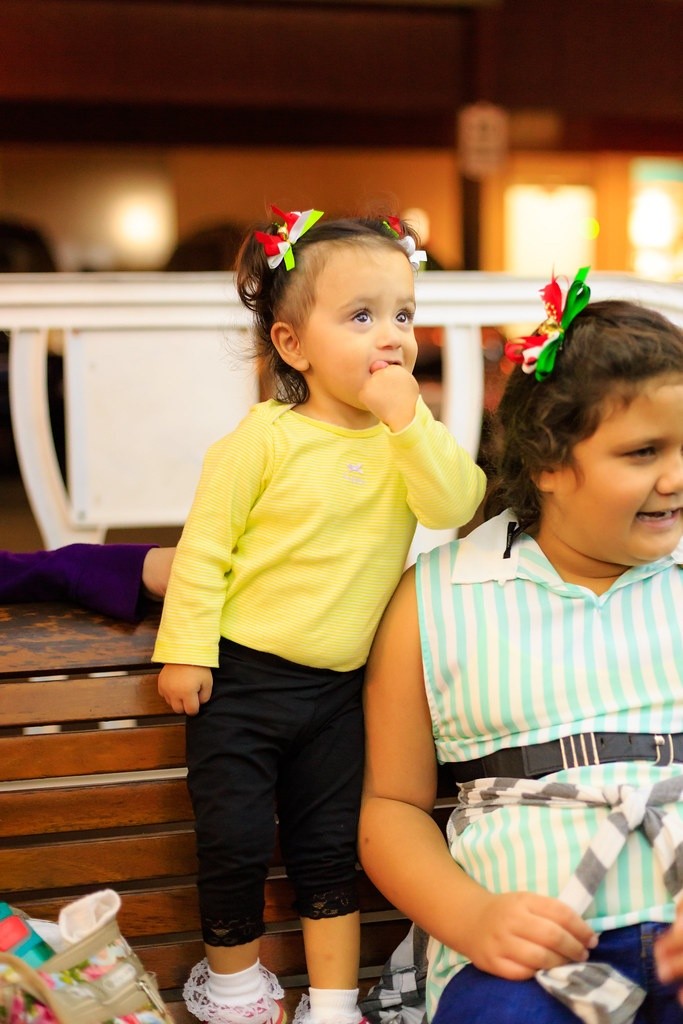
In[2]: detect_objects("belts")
[478,731,683,779]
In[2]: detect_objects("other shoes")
[203,999,288,1024]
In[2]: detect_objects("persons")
[151,205,488,1024]
[0,543,180,624]
[360,264,683,1024]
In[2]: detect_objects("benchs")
[0,603,419,1024]
